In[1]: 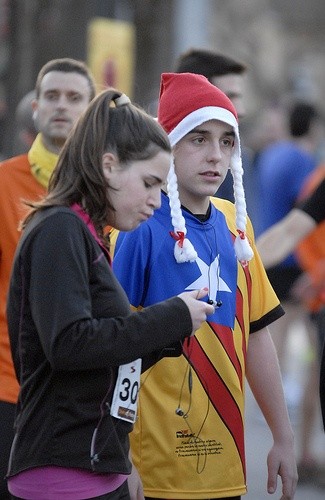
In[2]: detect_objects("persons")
[4,89,216,500]
[0,57,113,500]
[103,72,299,500]
[176,49,248,211]
[250,103,325,482]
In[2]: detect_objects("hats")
[156,72,255,264]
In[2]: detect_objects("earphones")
[208,300,213,305]
[217,301,223,307]
[176,409,183,416]
